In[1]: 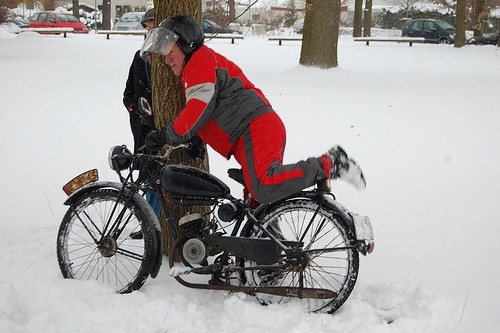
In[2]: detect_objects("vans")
[28,9,89,35]
[111,11,146,32]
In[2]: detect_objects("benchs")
[98,32,146,39]
[205,36,244,44]
[22,30,73,38]
[268,38,302,45]
[354,37,425,46]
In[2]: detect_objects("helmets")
[141,7,155,29]
[157,14,204,53]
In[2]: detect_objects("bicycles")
[54,125,376,316]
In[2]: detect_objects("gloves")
[182,135,206,163]
[131,109,155,130]
[144,123,179,151]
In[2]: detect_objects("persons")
[122,8,161,240]
[139,16,366,285]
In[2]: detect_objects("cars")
[401,18,456,45]
[200,18,243,36]
[3,18,29,35]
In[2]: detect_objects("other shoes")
[325,145,366,192]
[129,229,143,240]
[239,263,284,300]
